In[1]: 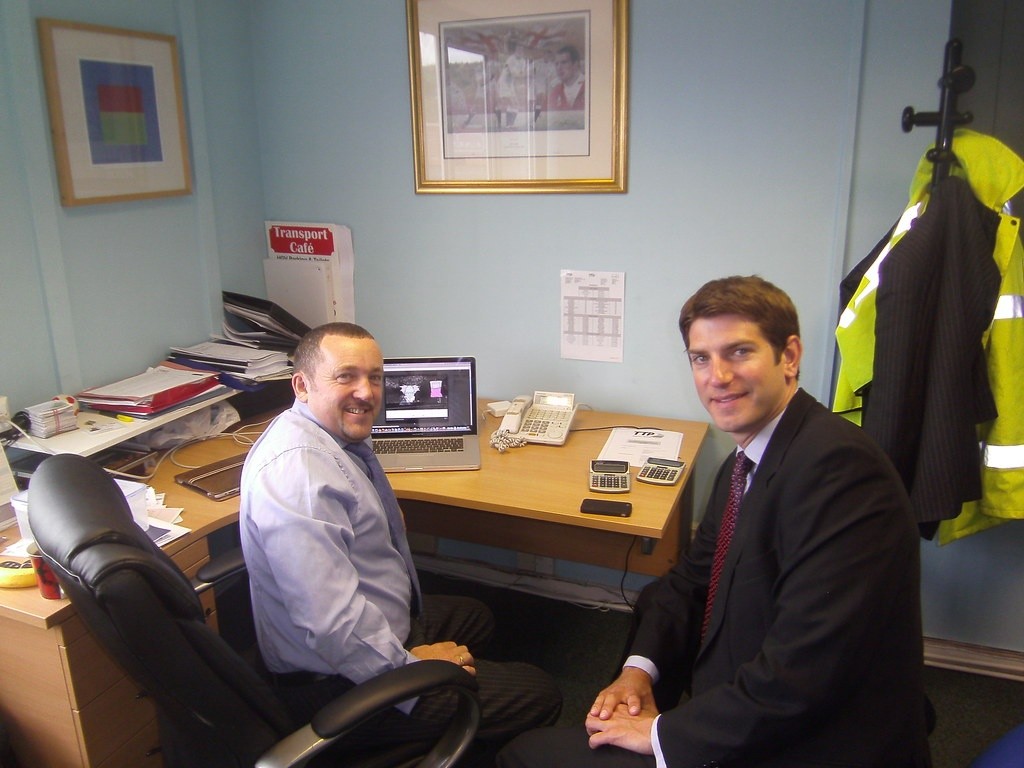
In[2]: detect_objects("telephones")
[498,390,578,446]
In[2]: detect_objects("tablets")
[175,451,250,502]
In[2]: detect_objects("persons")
[493,275,938,766]
[452,43,586,132]
[240,323,562,767]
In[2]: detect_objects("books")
[168,343,293,382]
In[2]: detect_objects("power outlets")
[516,553,553,576]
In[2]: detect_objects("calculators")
[637,456,686,486]
[589,459,630,493]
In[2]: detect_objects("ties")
[346,442,422,619]
[701,450,747,647]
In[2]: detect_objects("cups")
[26,542,64,600]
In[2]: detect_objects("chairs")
[27,454,481,768]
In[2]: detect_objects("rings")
[457,655,465,665]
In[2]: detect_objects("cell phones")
[580,499,632,518]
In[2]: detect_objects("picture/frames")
[35,19,194,207]
[405,1,628,193]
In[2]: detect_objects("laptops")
[370,357,483,473]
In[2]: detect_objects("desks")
[1,359,710,768]
